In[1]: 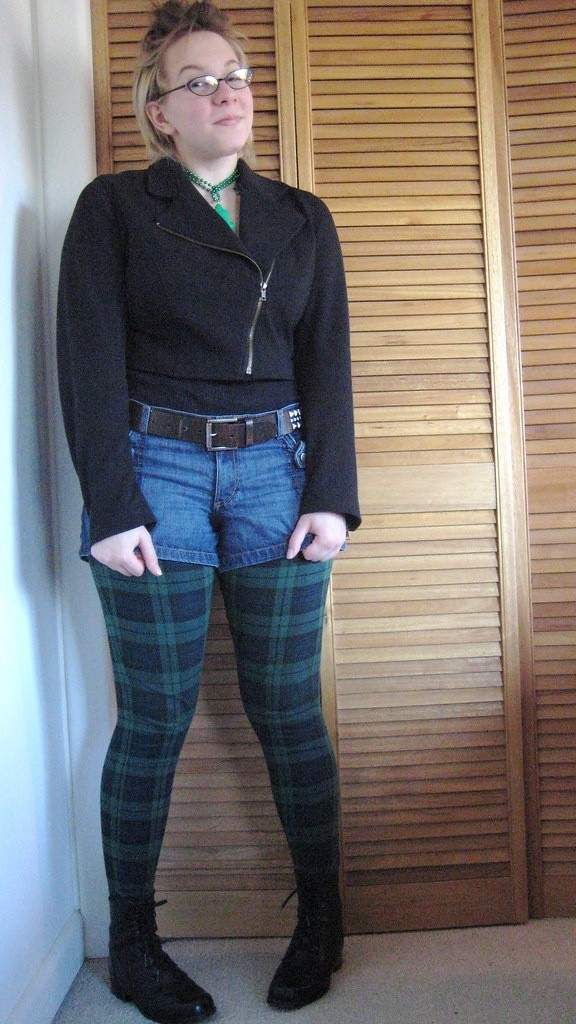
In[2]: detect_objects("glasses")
[154,68,255,101]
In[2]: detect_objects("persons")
[58,1,363,1024]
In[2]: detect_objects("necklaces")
[183,168,241,233]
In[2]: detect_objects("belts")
[130,402,303,451]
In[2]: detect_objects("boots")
[108,895,217,1024]
[264,887,345,1012]
